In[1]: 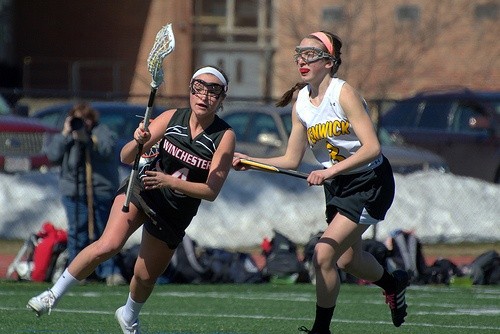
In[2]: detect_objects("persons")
[25,66,238,334]
[47,103,126,286]
[231,30,411,334]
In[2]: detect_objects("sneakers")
[114,305,141,334]
[26,290,55,318]
[382,270,409,328]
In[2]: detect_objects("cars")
[218,104,448,175]
[0,95,172,175]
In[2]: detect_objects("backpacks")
[4,223,499,285]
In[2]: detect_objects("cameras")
[70,118,84,130]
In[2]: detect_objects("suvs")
[380,85,500,183]
